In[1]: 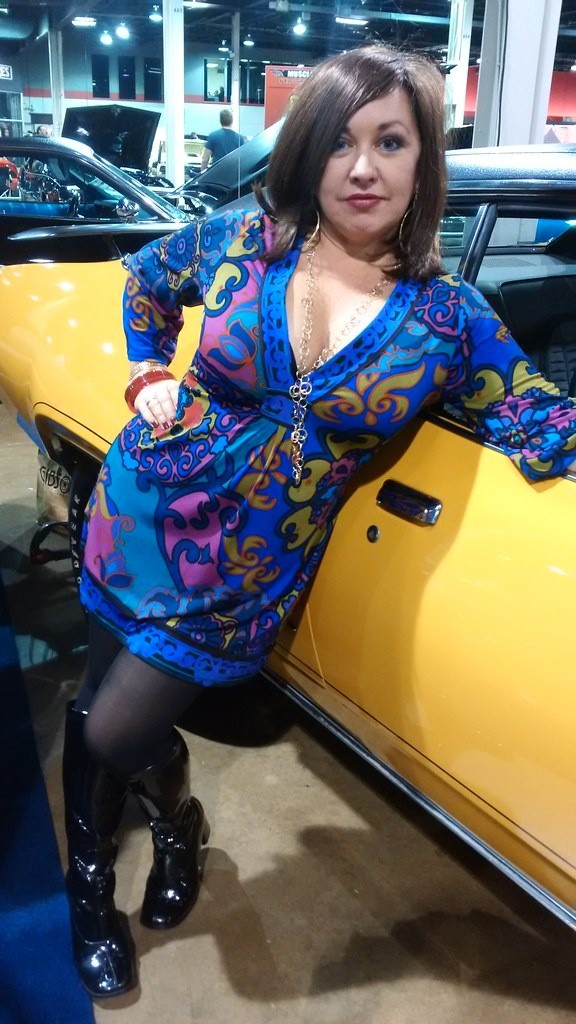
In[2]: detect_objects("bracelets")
[125,370,177,414]
[129,361,167,381]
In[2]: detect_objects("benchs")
[0,200,98,218]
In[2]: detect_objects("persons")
[201,109,243,171]
[37,126,47,136]
[60,47,576,998]
[208,86,224,102]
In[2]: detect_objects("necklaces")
[288,234,398,485]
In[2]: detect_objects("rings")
[146,398,156,406]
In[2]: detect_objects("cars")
[51,105,180,207]
[0,116,287,262]
[0,144,576,941]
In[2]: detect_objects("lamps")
[293,18,306,34]
[148,5,163,21]
[100,31,113,45]
[115,23,129,38]
[333,14,369,26]
[218,40,229,52]
[243,33,254,46]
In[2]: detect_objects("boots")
[128,728,210,931]
[62,700,136,998]
